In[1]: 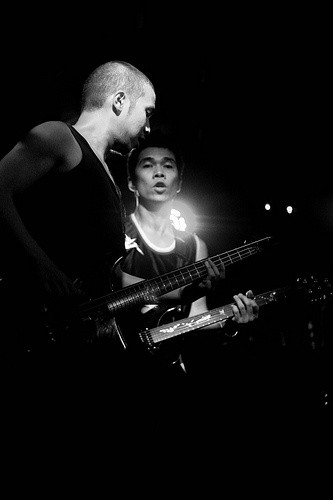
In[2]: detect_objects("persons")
[0,62,225,350]
[125,138,261,372]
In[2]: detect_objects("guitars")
[93,276,333,356]
[24,234,274,355]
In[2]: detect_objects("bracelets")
[220,323,239,339]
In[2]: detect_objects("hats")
[128,128,180,180]
